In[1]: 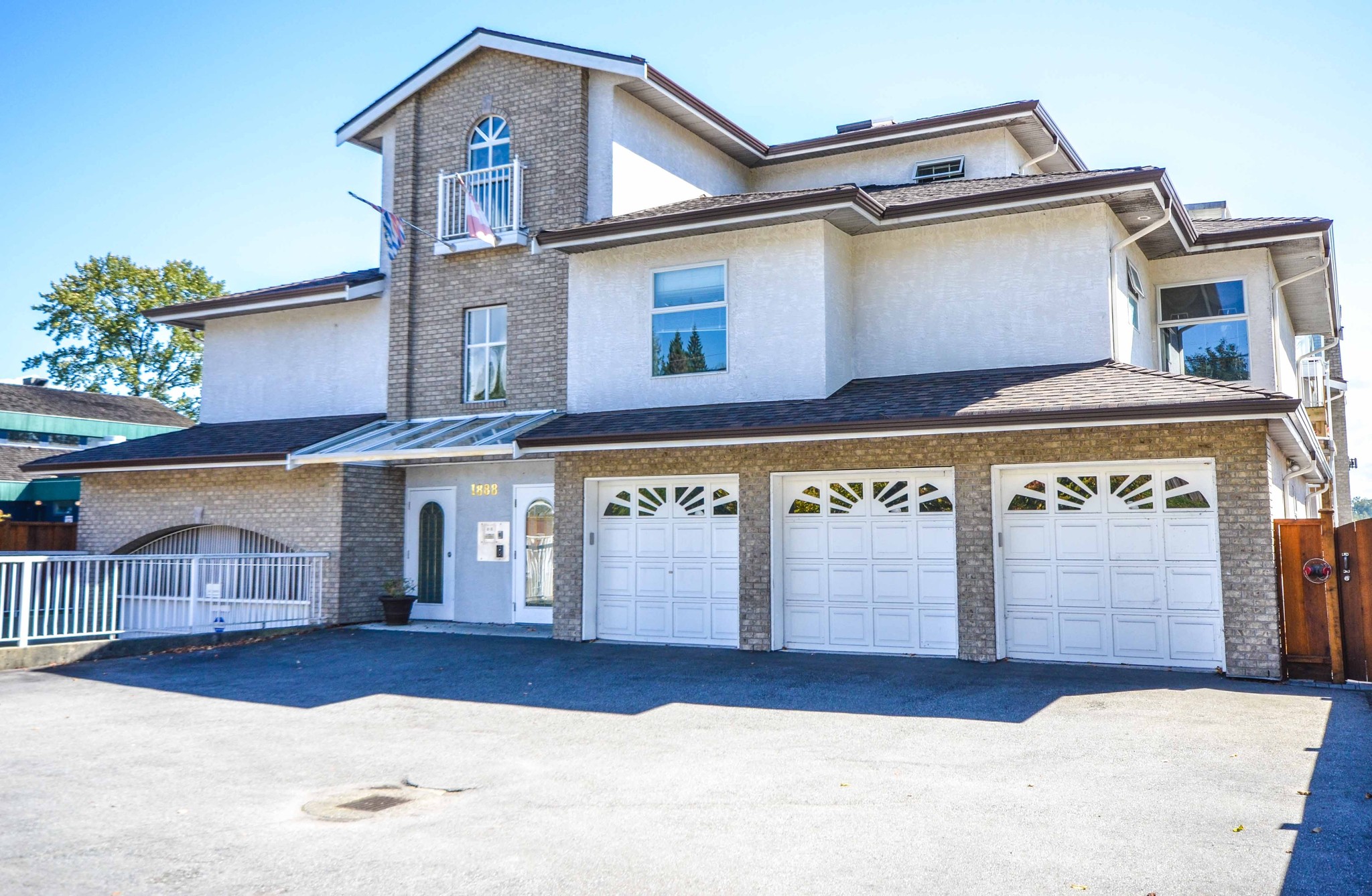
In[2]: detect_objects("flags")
[458,177,495,247]
[358,199,405,260]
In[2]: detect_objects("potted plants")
[378,578,418,625]
